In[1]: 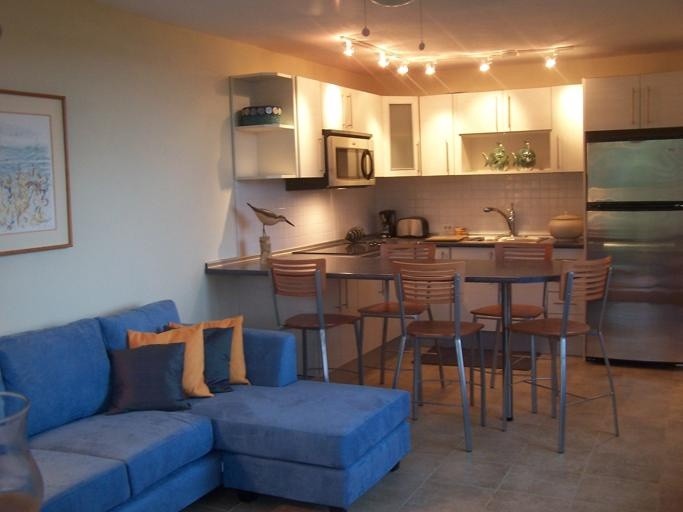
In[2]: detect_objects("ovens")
[396,215,429,238]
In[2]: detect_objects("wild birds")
[246,201,295,237]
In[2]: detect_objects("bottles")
[259,237,273,267]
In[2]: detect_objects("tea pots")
[481,142,511,172]
[507,141,535,172]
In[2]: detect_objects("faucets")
[482,203,516,236]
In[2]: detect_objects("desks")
[205,250,586,421]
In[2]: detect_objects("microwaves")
[285,129,377,190]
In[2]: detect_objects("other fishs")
[343,223,369,244]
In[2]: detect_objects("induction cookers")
[291,241,385,256]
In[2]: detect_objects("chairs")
[391,260,487,453]
[469,240,555,414]
[501,256,619,454]
[358,242,445,392]
[266,258,360,384]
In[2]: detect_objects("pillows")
[106,315,251,415]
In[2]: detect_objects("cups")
[0,392,45,511]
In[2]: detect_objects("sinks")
[460,235,550,245]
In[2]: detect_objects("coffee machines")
[374,210,398,239]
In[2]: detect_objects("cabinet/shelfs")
[582,70,683,132]
[419,84,586,177]
[230,72,299,181]
[383,95,422,177]
[294,75,382,178]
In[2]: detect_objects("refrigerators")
[583,126,682,368]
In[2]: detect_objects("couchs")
[1,297,413,512]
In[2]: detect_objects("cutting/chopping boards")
[424,234,466,242]
